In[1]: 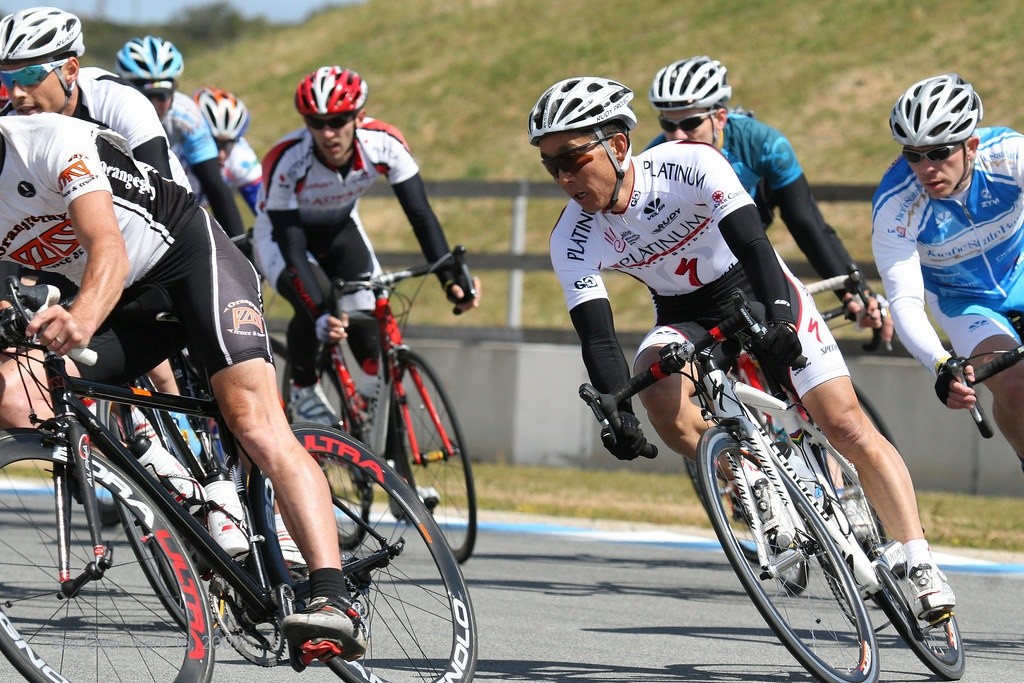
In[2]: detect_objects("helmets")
[0,7,86,58]
[114,35,184,89]
[889,73,983,148]
[192,86,250,140]
[294,66,368,118]
[528,77,638,147]
[648,56,732,110]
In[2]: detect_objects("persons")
[252,64,481,521]
[870,72,1024,471]
[642,55,895,342]
[528,78,956,619]
[0,7,371,661]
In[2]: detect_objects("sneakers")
[741,472,796,554]
[904,560,955,617]
[389,485,440,520]
[287,374,339,430]
[282,595,367,662]
[165,477,216,579]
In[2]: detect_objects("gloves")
[601,409,646,460]
[752,320,802,367]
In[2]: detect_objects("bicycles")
[577,273,1023,683]
[0,278,482,683]
[280,246,481,566]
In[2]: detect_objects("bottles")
[204,466,249,561]
[774,442,830,511]
[355,357,378,421]
[127,434,207,515]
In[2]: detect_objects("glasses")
[657,109,717,133]
[305,110,357,130]
[144,93,171,102]
[0,58,69,88]
[902,142,962,164]
[541,131,626,179]
[213,138,234,153]
